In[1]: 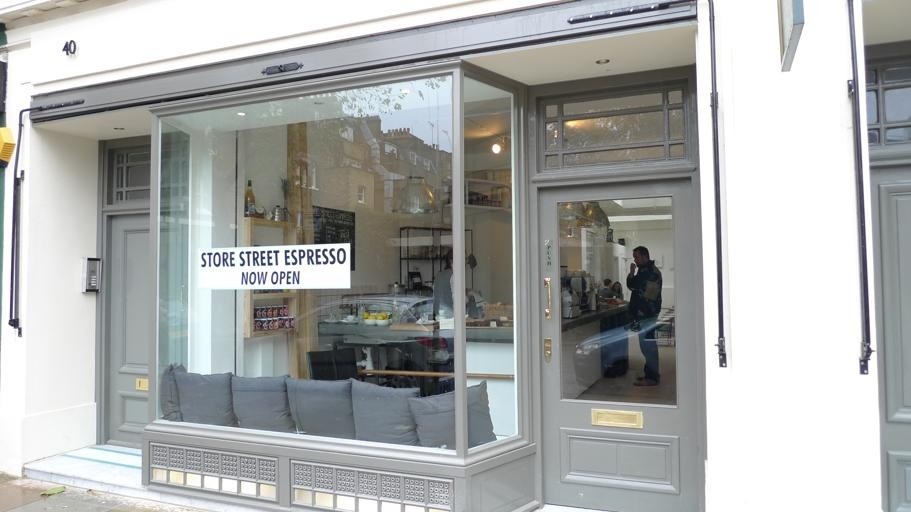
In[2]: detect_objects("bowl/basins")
[362,317,392,327]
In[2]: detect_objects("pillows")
[286,377,355,440]
[175,371,238,426]
[349,379,419,445]
[230,376,294,433]
[408,381,496,450]
[161,365,191,423]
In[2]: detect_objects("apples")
[364,312,388,320]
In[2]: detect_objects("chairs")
[305,337,457,397]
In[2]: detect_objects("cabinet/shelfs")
[442,175,509,212]
[399,226,477,298]
[242,216,297,341]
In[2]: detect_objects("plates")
[323,317,359,324]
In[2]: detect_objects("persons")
[611,281,624,300]
[625,245,663,386]
[432,247,475,392]
[598,277,619,299]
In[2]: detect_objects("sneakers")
[633,375,659,386]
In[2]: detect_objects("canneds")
[255,305,295,331]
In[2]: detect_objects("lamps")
[390,172,438,216]
[557,200,612,229]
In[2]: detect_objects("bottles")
[244,178,256,217]
[272,205,283,222]
